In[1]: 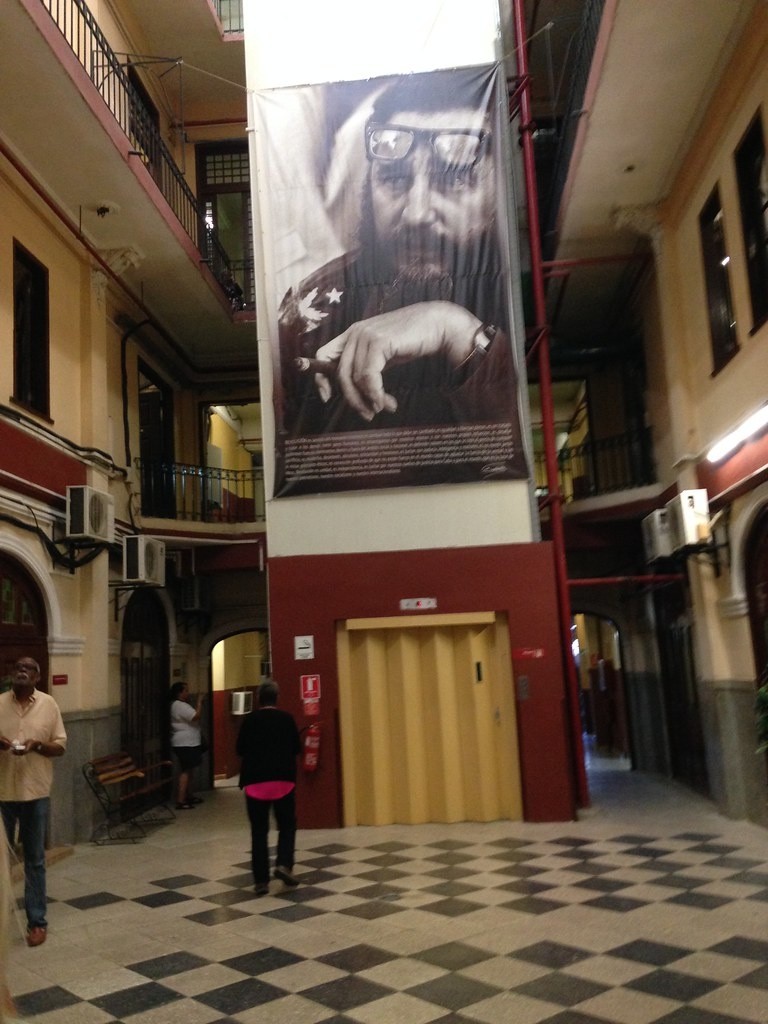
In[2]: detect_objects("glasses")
[15,663,38,672]
[366,122,493,172]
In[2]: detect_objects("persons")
[273,68,526,497]
[235,682,305,895]
[168,682,205,810]
[0,657,67,947]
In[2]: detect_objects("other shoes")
[274,865,299,886]
[254,884,268,895]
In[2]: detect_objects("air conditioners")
[641,507,672,564]
[122,535,166,587]
[65,485,116,545]
[666,489,713,553]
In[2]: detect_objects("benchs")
[82,748,177,845]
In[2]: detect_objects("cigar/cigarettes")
[294,357,337,373]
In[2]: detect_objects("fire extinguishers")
[303,719,323,775]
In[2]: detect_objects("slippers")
[187,797,202,804]
[175,802,194,809]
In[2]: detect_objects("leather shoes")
[27,926,46,946]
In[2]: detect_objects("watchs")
[445,323,495,384]
